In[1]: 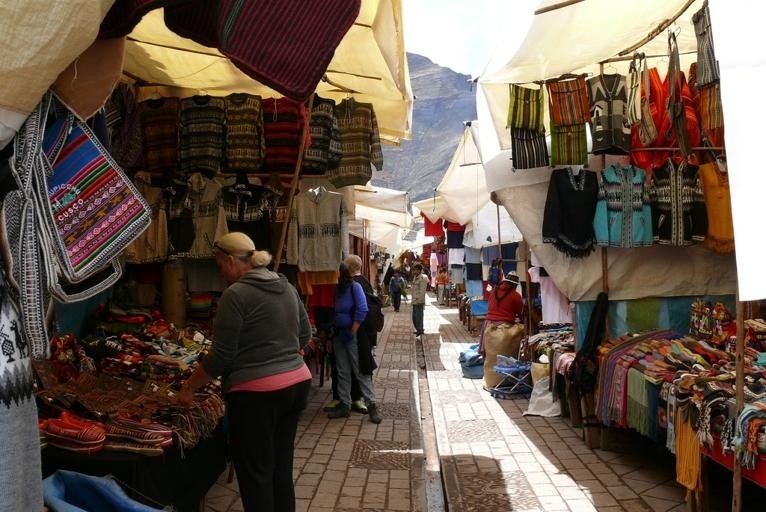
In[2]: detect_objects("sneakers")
[38,410,174,457]
[413,331,423,337]
[323,399,382,424]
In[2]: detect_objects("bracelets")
[182,381,200,395]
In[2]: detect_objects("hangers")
[300,174,340,198]
[128,82,355,107]
[504,0,726,177]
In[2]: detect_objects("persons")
[388,269,406,312]
[176,232,313,511]
[434,269,448,305]
[326,264,384,425]
[322,254,382,414]
[479,271,525,359]
[410,264,430,337]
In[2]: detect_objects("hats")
[503,273,520,285]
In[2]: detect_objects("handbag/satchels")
[351,304,378,327]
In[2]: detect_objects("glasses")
[211,241,231,255]
